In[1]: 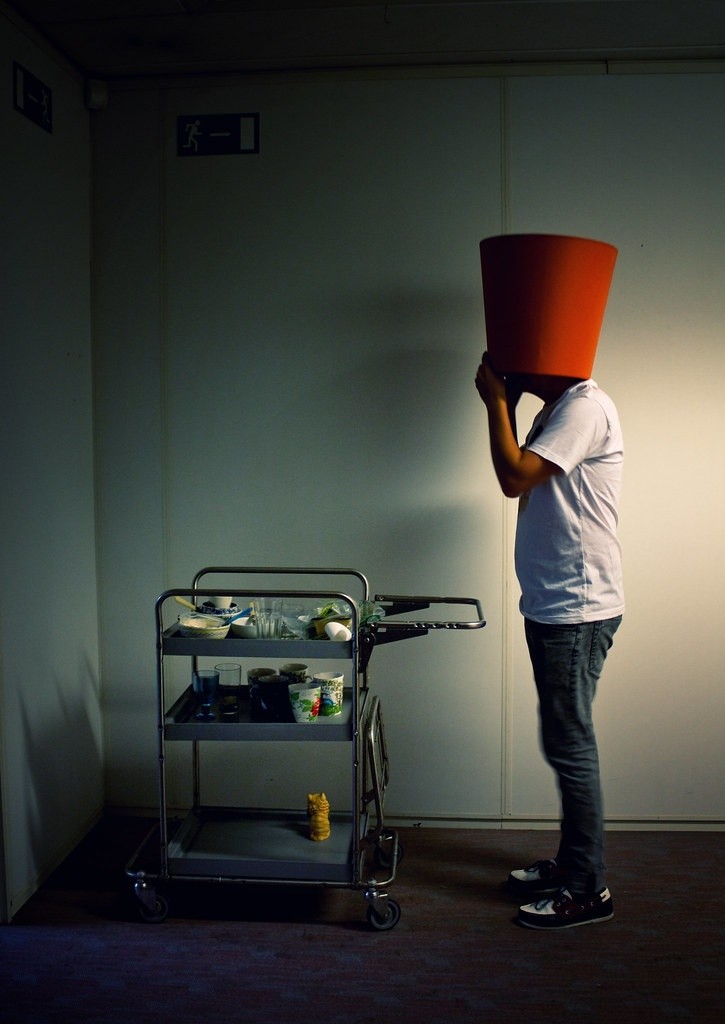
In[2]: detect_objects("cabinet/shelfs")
[117,562,486,932]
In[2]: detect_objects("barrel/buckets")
[479,235,619,382]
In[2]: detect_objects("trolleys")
[126,566,481,931]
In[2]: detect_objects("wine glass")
[191,669,220,720]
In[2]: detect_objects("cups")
[247,667,277,704]
[288,682,321,723]
[278,663,312,685]
[258,674,291,719]
[253,597,281,640]
[280,597,303,640]
[214,663,242,718]
[313,671,344,716]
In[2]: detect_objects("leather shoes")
[518,886,615,931]
[509,858,565,885]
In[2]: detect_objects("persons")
[474,351,626,930]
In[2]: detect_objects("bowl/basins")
[178,619,231,639]
[202,601,238,615]
[231,617,275,638]
[208,596,232,610]
[191,607,241,622]
